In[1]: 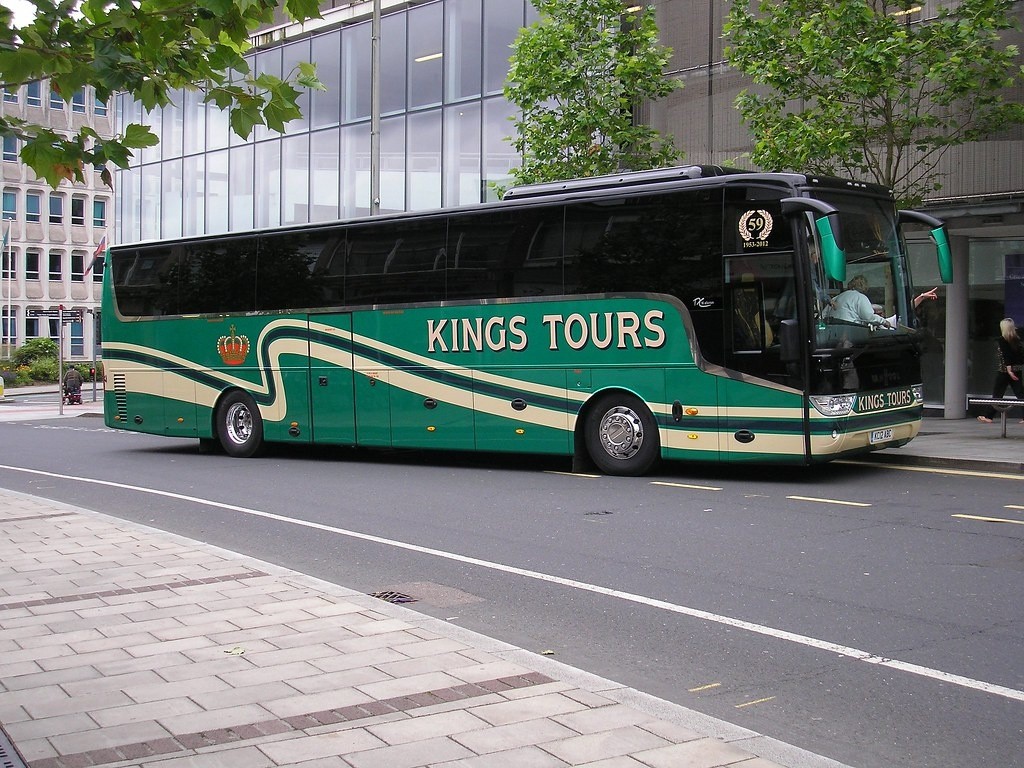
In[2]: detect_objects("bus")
[100,164,954,477]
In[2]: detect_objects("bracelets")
[920,293,925,300]
[1008,372,1014,375]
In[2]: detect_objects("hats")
[69,365,74,368]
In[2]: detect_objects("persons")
[819,275,891,330]
[976,318,1024,424]
[871,287,938,330]
[773,235,838,323]
[63,365,84,395]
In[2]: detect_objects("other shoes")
[1019,418,1024,424]
[977,416,993,423]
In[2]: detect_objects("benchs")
[969,399,1024,438]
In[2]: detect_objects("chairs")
[733,287,781,349]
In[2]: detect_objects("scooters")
[63,385,83,405]
